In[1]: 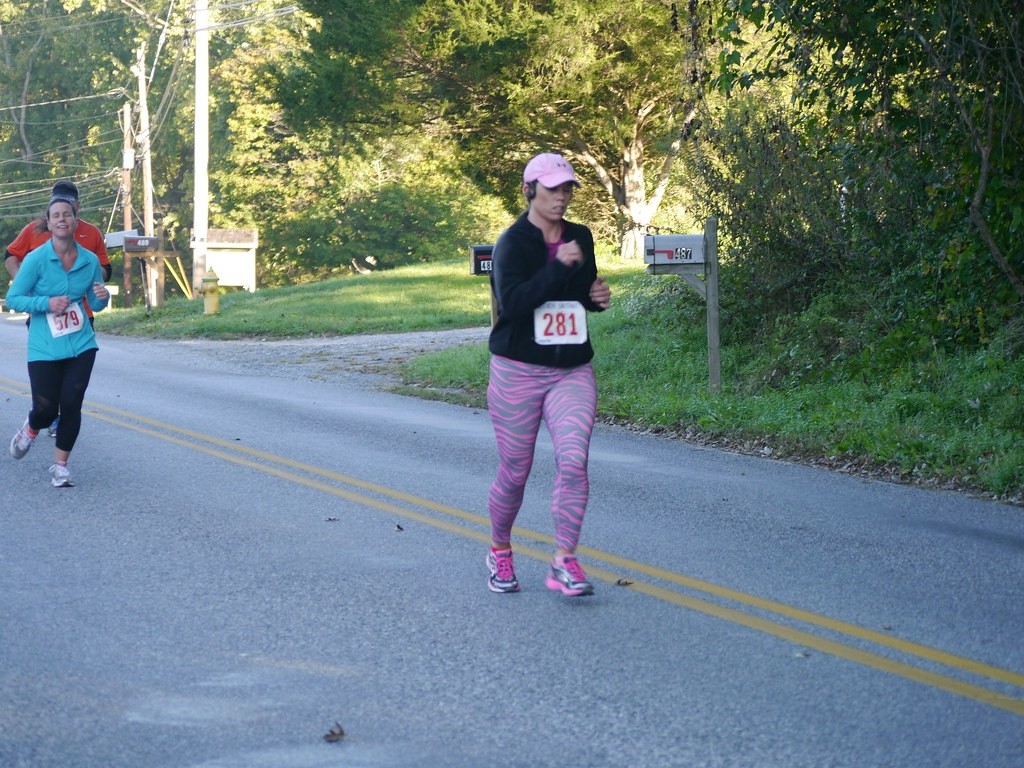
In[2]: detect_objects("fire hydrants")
[193,266,220,316]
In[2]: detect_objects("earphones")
[525,182,535,198]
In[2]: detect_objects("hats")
[52,180,78,201]
[523,153,581,189]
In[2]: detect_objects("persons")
[5,199,110,487]
[486,152,612,596]
[5,180,113,439]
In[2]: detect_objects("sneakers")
[486,545,519,593]
[10,416,39,459]
[546,552,595,595]
[48,413,60,437]
[48,461,75,487]
